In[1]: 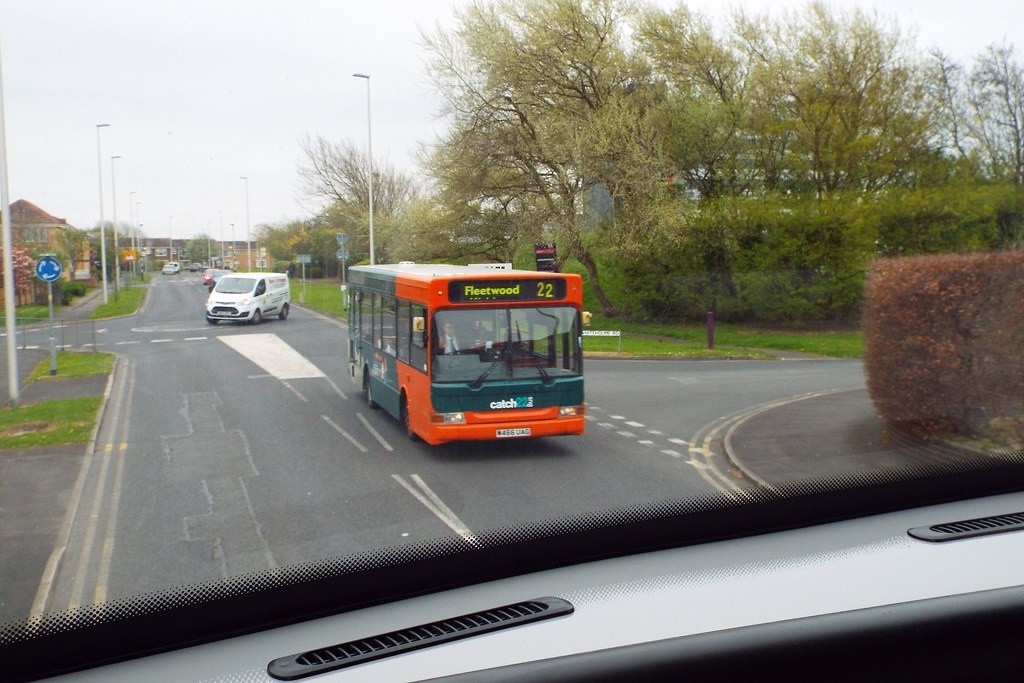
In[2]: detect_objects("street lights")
[353,73,375,266]
[97,123,111,303]
[217,210,225,271]
[240,176,252,272]
[110,155,121,294]
[130,190,144,280]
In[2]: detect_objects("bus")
[346,262,593,445]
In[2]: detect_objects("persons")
[441,322,468,356]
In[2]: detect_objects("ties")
[450,339,457,353]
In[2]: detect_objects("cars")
[202,269,232,288]
[168,262,182,275]
[189,263,202,272]
[162,265,175,276]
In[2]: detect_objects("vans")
[205,272,290,326]
